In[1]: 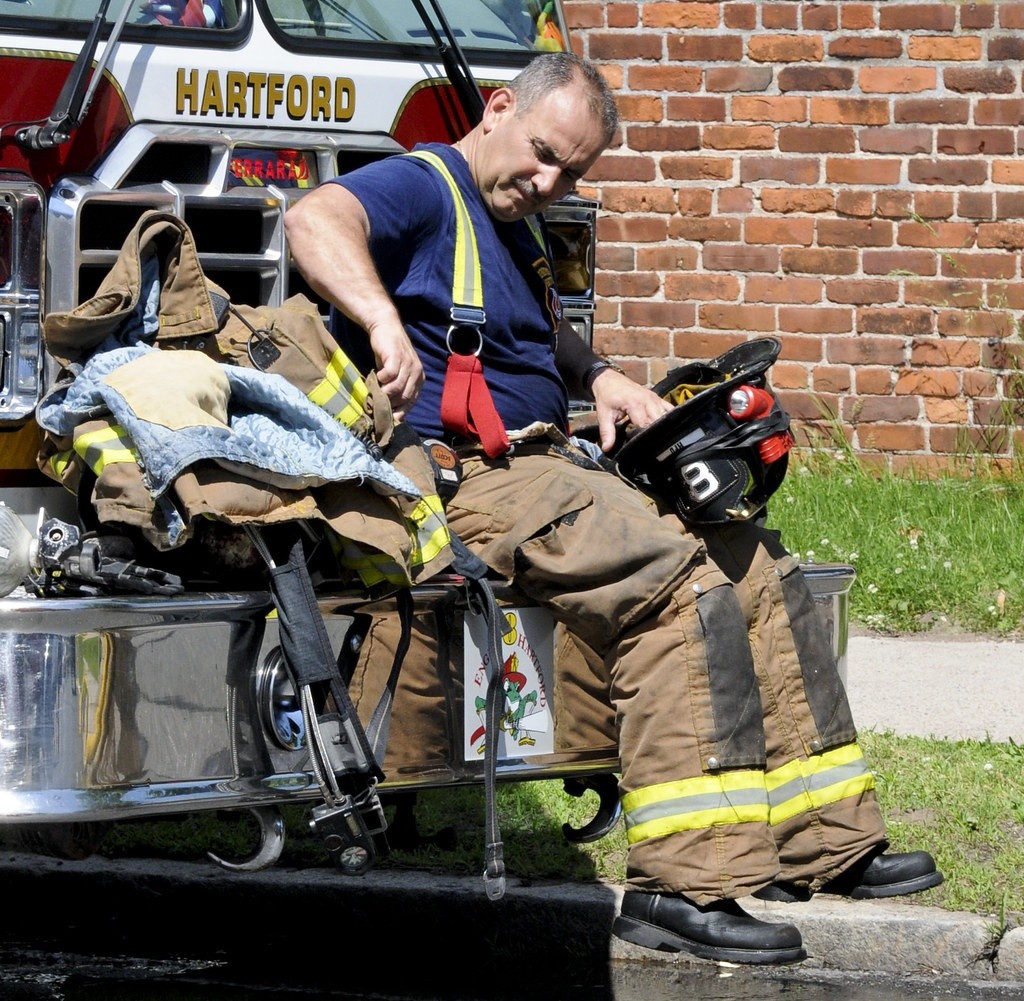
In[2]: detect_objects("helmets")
[594,337,794,522]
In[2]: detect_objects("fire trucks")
[1,1,857,824]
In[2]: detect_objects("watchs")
[583,360,625,395]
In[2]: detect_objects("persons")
[284,50,953,961]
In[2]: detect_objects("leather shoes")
[611,889,806,965]
[821,849,946,898]
